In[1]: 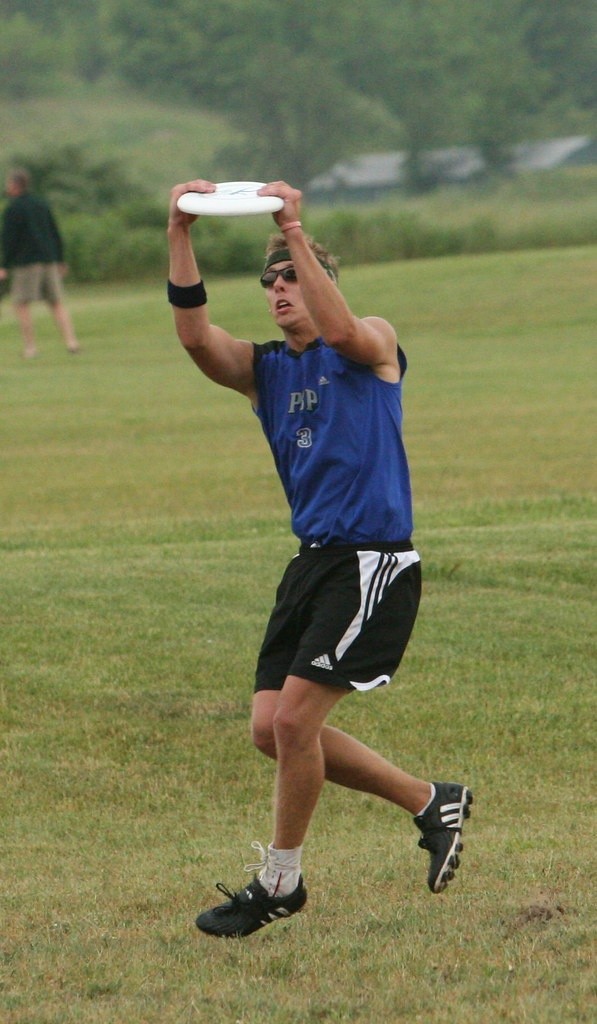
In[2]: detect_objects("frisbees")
[178,182,283,217]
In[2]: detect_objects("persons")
[168,180,474,940]
[0,173,83,358]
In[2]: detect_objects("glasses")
[261,266,297,289]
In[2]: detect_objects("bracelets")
[168,276,208,309]
[279,220,302,232]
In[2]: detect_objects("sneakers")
[414,782,473,893]
[196,874,308,937]
